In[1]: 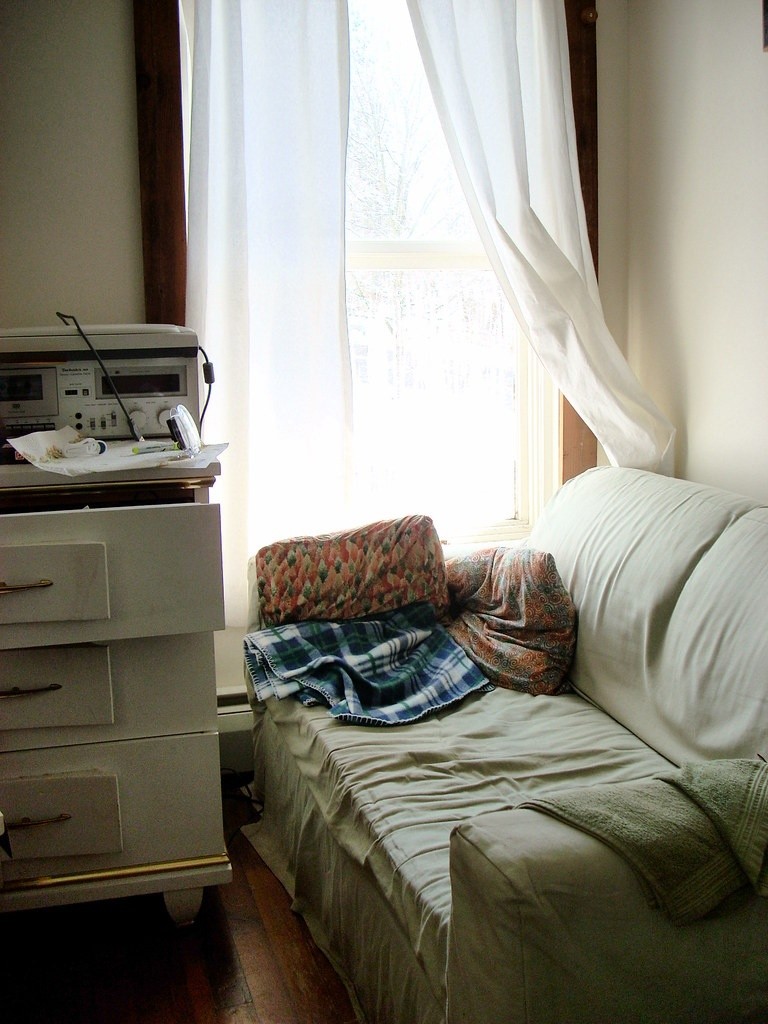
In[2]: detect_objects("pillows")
[448,546,580,696]
[258,516,447,625]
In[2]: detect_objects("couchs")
[252,467,768,1024]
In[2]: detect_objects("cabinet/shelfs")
[0,438,236,929]
